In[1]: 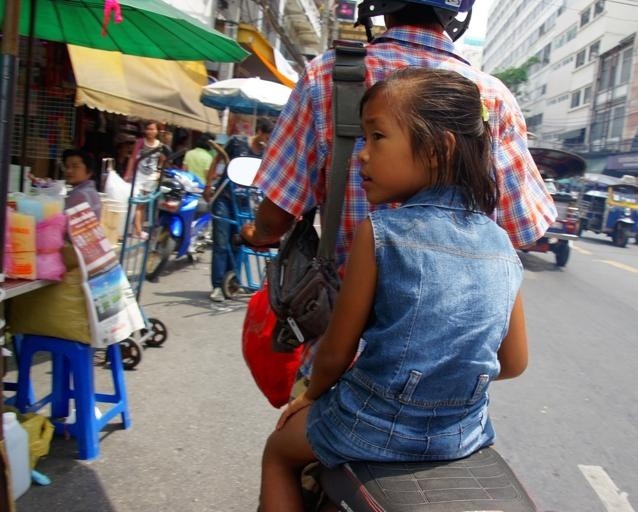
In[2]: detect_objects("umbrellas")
[0,0,252,196]
[198,74,292,138]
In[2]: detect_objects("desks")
[0,244,119,302]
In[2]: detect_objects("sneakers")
[210,288,226,304]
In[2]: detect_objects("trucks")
[524,136,585,270]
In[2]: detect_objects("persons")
[123,119,228,236]
[55,146,100,222]
[254,63,534,511]
[202,118,276,304]
[229,1,565,337]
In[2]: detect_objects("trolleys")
[102,144,178,372]
[209,140,280,298]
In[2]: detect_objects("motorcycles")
[578,172,637,247]
[146,169,212,283]
[227,158,540,512]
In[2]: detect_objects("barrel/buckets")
[2,411,33,502]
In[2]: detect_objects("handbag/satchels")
[262,219,343,352]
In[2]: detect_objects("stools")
[15,335,131,460]
[2,333,36,414]
